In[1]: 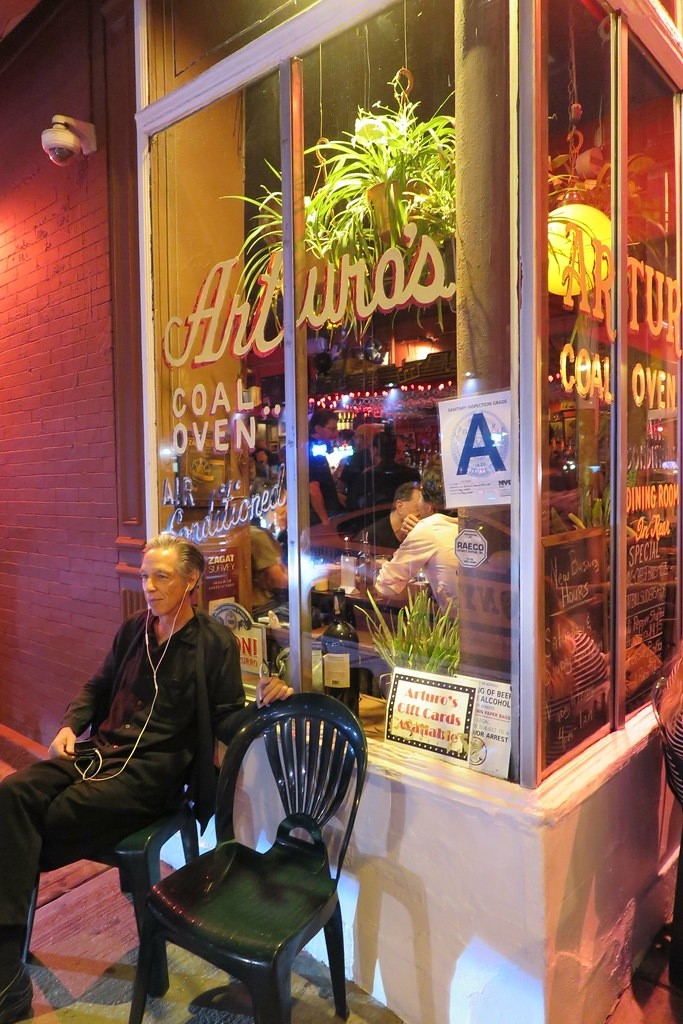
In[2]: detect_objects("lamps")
[544,202,613,297]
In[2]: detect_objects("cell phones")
[74,741,101,754]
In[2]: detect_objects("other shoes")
[0,962,33,1024]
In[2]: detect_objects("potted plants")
[216,71,457,349]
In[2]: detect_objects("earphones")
[186,581,190,591]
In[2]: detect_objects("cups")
[373,555,390,589]
[327,564,341,590]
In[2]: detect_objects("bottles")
[321,588,359,718]
[356,540,365,567]
[358,541,373,598]
[343,537,352,557]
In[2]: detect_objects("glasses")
[323,426,336,433]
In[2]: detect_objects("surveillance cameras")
[42,128,81,167]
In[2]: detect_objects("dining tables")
[268,562,436,621]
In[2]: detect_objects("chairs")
[126,692,368,1024]
[652,674,682,808]
[18,721,221,996]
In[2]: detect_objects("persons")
[657,640,683,806]
[542,580,611,771]
[0,534,294,1024]
[245,407,458,647]
